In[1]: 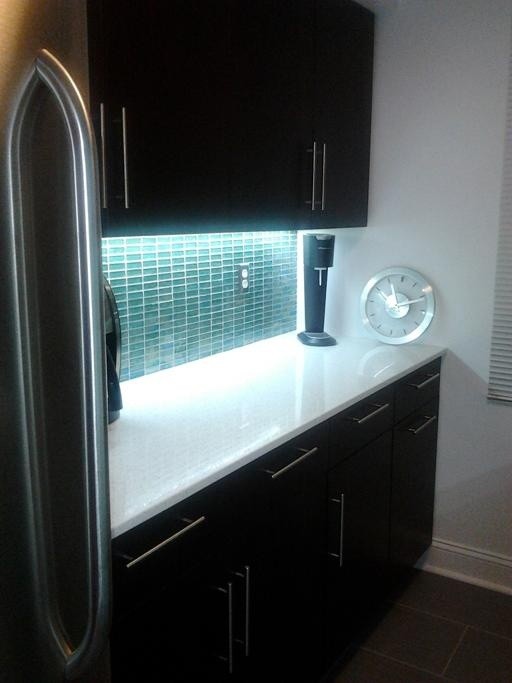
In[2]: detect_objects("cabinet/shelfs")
[86,0,375,237]
[112,357,441,683]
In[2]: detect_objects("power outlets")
[238,264,249,293]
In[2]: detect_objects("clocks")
[360,267,435,345]
